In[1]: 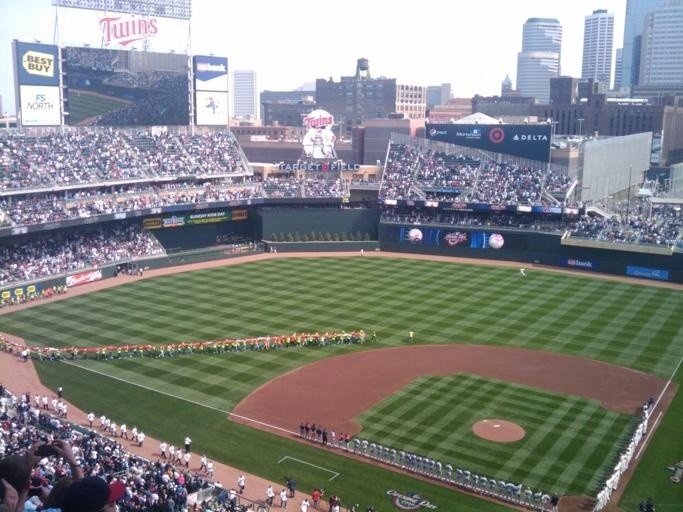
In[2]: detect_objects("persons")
[350,177,381,183]
[520,267,525,276]
[1,386,560,512]
[0,327,380,362]
[407,330,414,344]
[360,249,363,257]
[381,143,682,249]
[1,121,352,305]
[594,397,654,512]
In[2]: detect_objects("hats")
[59,474,127,512]
[0,452,42,496]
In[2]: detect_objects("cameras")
[37,444,64,456]
